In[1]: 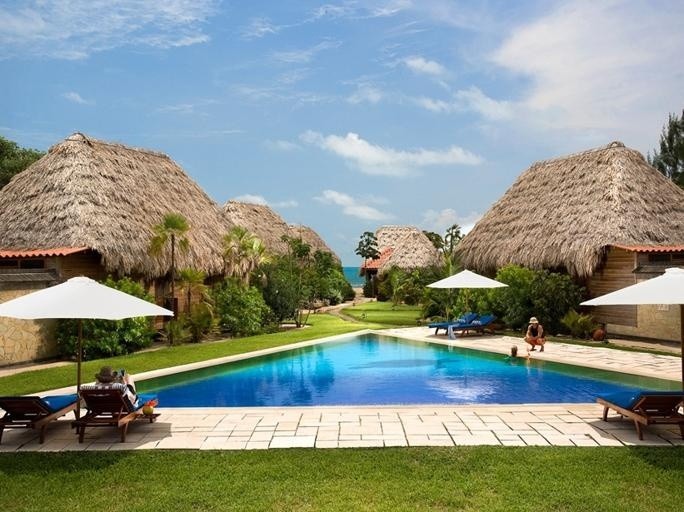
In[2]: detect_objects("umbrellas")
[579,267,683,392]
[0,275,174,434]
[425,269,510,334]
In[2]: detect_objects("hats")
[528,316,538,324]
[95,366,116,381]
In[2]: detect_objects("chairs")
[596,388,684,441]
[0,388,158,445]
[427,312,497,338]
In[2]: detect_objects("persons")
[94,365,138,405]
[523,317,546,352]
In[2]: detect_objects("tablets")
[111,369,126,379]
